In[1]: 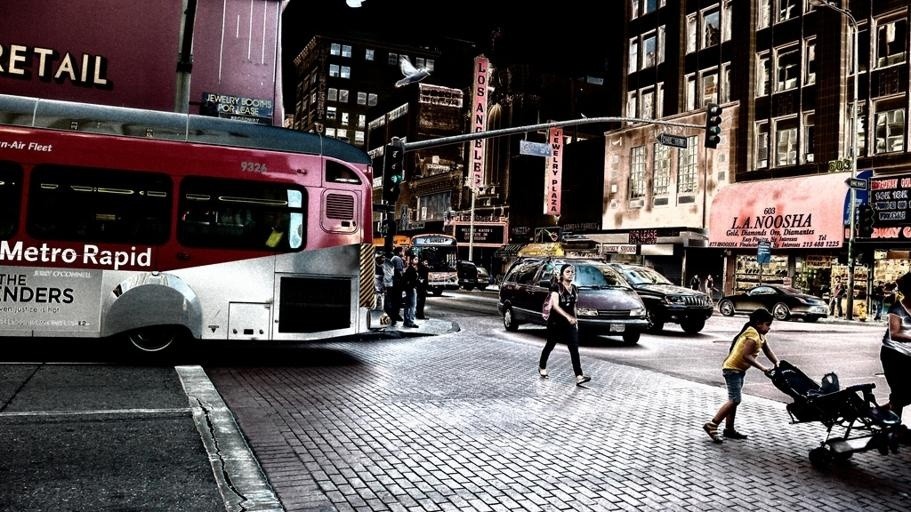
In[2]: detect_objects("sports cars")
[719,285,832,324]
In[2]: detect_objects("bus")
[0,92,377,360]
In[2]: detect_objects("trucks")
[373,235,461,295]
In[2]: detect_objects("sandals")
[703,419,723,442]
[724,424,747,439]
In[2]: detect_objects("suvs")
[458,260,478,289]
[613,262,715,332]
[476,266,491,290]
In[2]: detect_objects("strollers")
[770,355,904,466]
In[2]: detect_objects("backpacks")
[541,283,560,321]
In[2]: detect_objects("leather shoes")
[576,376,591,384]
[538,368,549,379]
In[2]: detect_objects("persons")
[705,275,720,305]
[537,264,591,386]
[704,309,782,444]
[831,275,846,317]
[376,244,432,331]
[881,270,911,425]
[690,274,702,293]
[872,280,884,322]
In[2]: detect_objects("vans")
[497,256,651,347]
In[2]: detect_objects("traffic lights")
[709,105,722,147]
[385,149,403,192]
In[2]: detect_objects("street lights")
[806,0,860,323]
